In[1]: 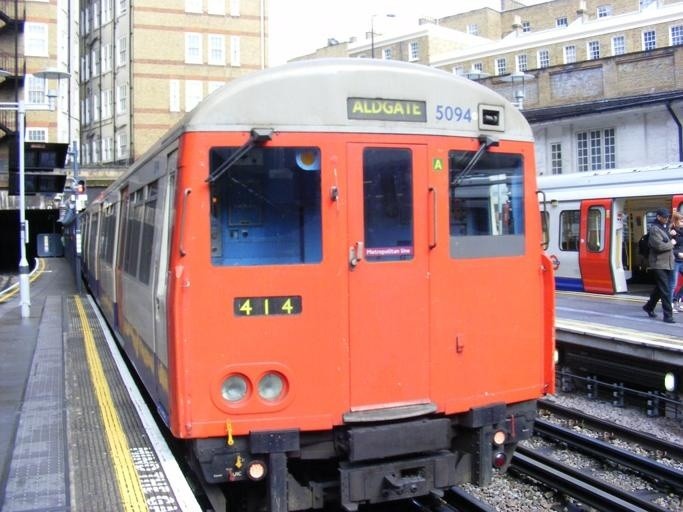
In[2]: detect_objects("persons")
[642,207,678,323]
[663,214,682,314]
[564,228,574,250]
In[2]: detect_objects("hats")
[655,207,671,217]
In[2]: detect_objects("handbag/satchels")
[638,232,651,257]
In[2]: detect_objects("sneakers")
[662,303,683,323]
[641,305,659,318]
